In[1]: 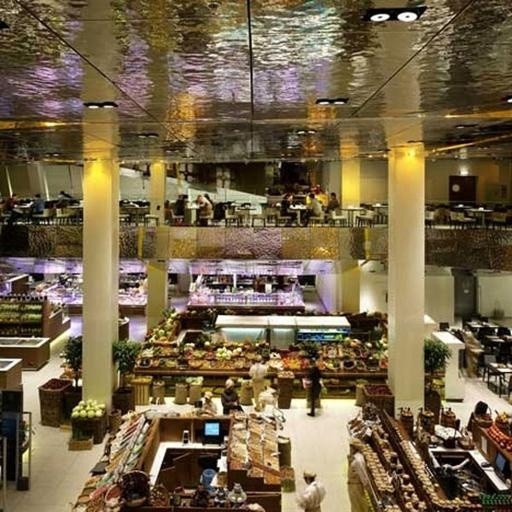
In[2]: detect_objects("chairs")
[438,313,512,401]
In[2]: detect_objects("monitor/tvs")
[202,420,222,445]
[495,453,508,472]
[444,471,460,496]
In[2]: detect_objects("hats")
[303,469,317,478]
[205,391,213,400]
[225,378,235,390]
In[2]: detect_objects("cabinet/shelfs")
[67,405,283,512]
[31,283,169,316]
[187,290,306,316]
[0,293,71,342]
[346,408,512,512]
[136,340,390,399]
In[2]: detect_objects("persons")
[54,191,72,204]
[295,469,325,512]
[279,184,338,228]
[5,193,20,226]
[221,379,239,414]
[346,439,372,512]
[173,193,215,227]
[249,355,266,412]
[306,359,321,417]
[30,194,45,224]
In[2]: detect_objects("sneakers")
[307,413,314,416]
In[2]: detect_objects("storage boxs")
[424,337,453,422]
[58,336,84,416]
[112,339,142,416]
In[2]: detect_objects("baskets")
[473,403,493,427]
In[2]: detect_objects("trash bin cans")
[361,384,394,418]
[38,378,73,428]
